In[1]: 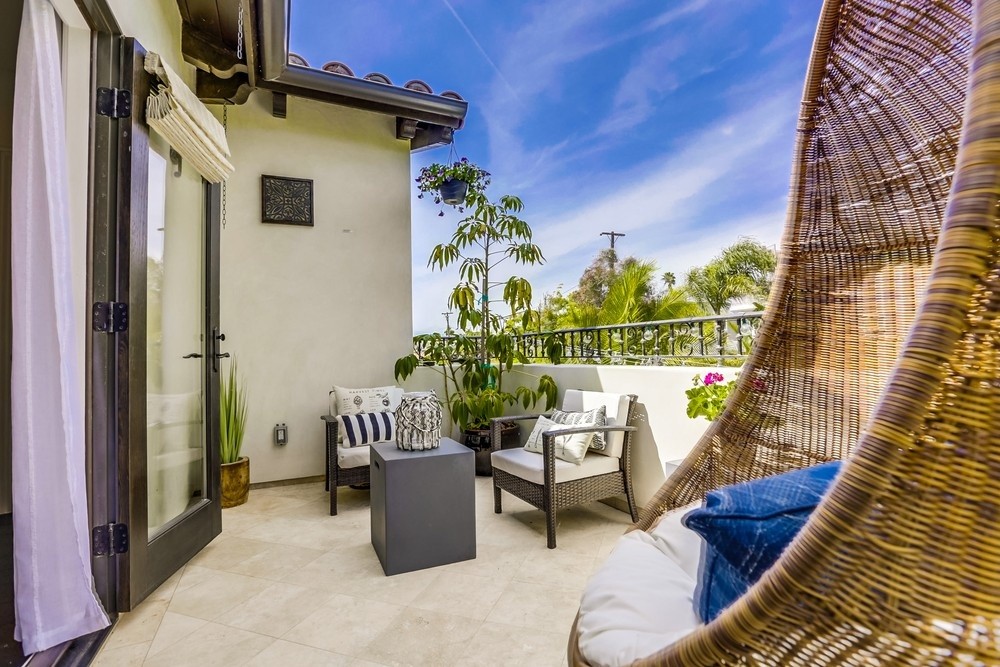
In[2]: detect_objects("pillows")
[524,405,609,465]
[680,462,844,625]
[333,386,397,449]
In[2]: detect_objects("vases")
[439,177,470,205]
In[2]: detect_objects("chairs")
[570,0,1000,667]
[320,391,370,518]
[490,387,638,548]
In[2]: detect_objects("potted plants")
[393,187,567,478]
[219,353,251,509]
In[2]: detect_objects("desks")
[369,438,481,579]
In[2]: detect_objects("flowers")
[415,156,492,217]
[685,371,786,429]
